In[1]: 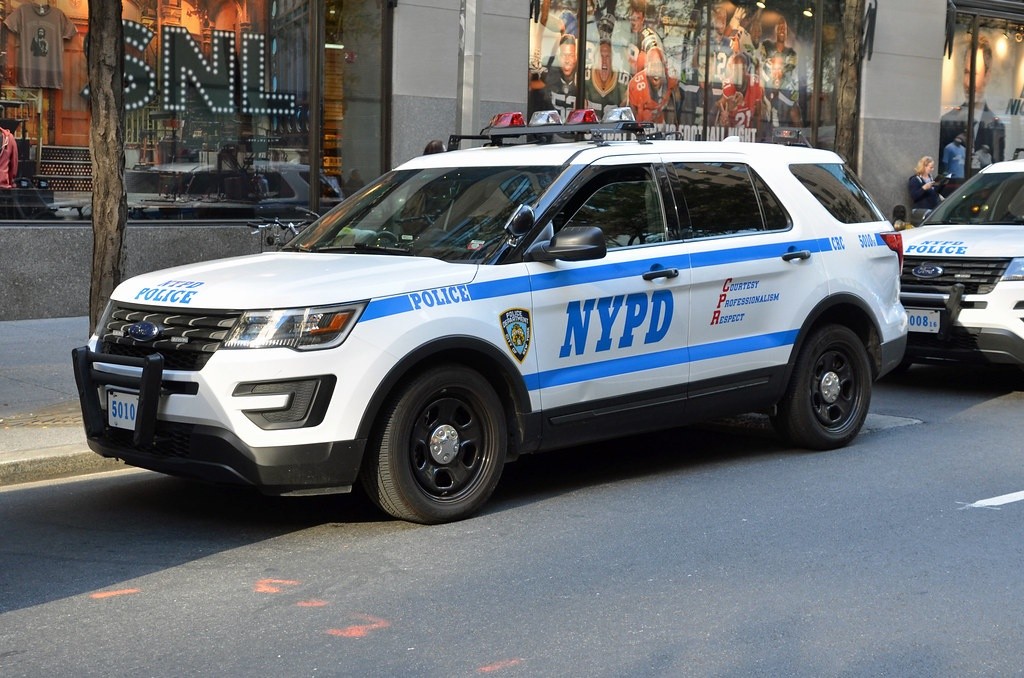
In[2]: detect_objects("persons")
[344,168,366,201]
[891,204,907,231]
[0,105,18,188]
[248,175,269,201]
[941,127,992,178]
[939,34,1005,166]
[529,0,804,141]
[908,156,946,210]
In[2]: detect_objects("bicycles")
[246,207,443,251]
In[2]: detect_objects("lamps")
[755,0,766,8]
[802,7,812,17]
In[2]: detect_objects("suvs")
[897,158,1024,367]
[71,107,910,525]
[44,161,343,217]
